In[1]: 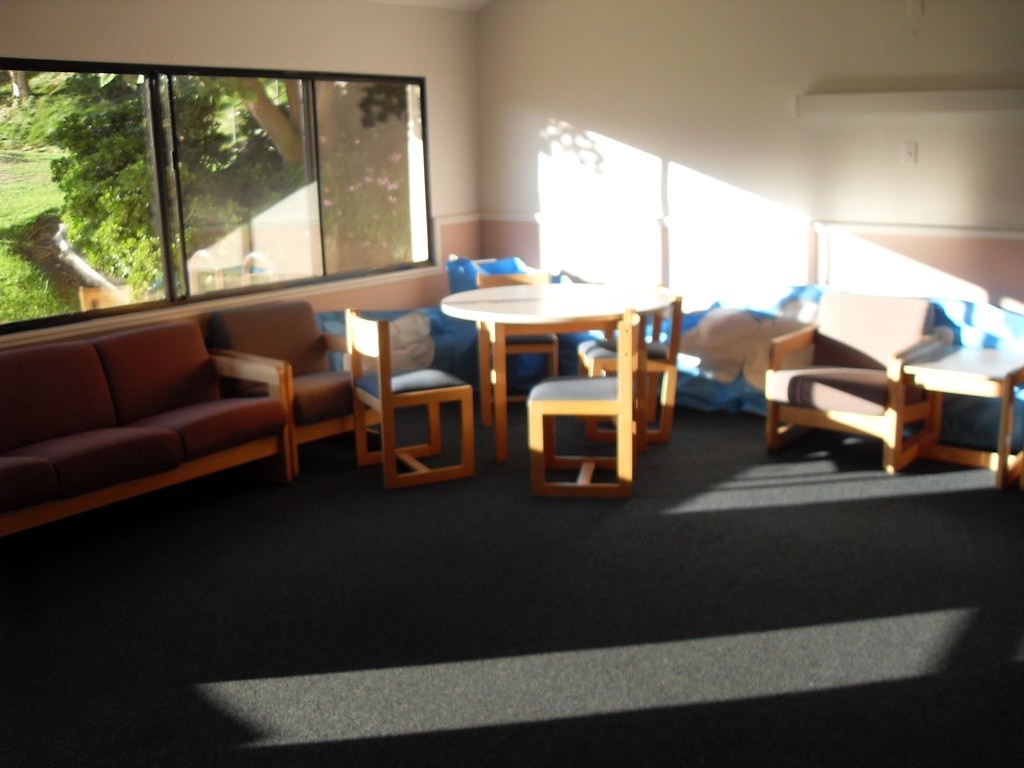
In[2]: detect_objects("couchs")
[0,319,293,538]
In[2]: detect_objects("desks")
[439,285,678,463]
[887,341,1024,489]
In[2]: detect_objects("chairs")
[477,273,558,403]
[763,289,953,473]
[578,297,682,443]
[344,308,476,488]
[208,298,381,479]
[526,310,646,497]
